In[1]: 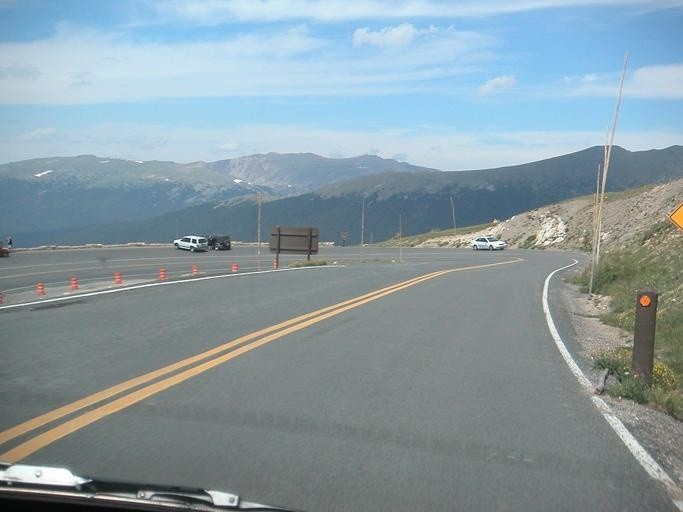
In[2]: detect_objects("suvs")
[174,235,232,252]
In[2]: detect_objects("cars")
[470,235,507,251]
[0,240,10,256]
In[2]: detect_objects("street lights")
[232,179,261,256]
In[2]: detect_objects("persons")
[8,238,12,248]
